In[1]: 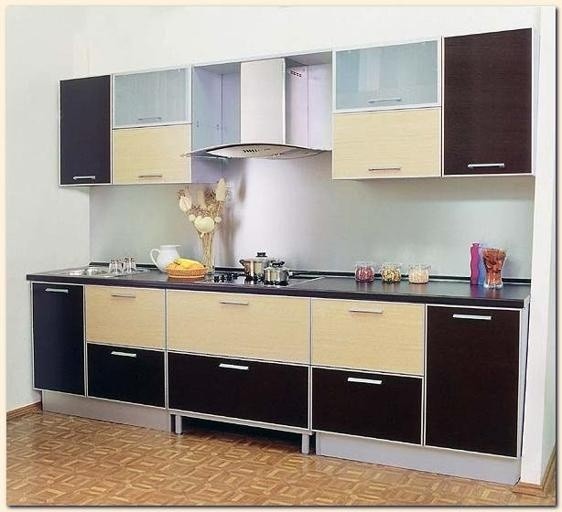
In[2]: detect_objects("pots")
[240,253,277,278]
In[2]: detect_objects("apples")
[167,261,203,269]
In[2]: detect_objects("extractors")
[178,58,332,162]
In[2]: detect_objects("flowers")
[197,228,216,275]
[178,179,228,231]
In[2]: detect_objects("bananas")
[174,258,200,265]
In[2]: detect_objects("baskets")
[166,267,207,278]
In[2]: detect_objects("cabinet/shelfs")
[330,25,535,177]
[58,67,194,187]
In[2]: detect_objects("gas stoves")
[194,273,323,289]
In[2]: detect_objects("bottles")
[407,265,431,283]
[381,263,400,281]
[469,242,506,289]
[354,261,375,282]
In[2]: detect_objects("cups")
[108,257,136,275]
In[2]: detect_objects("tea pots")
[150,244,181,273]
[264,261,289,282]
